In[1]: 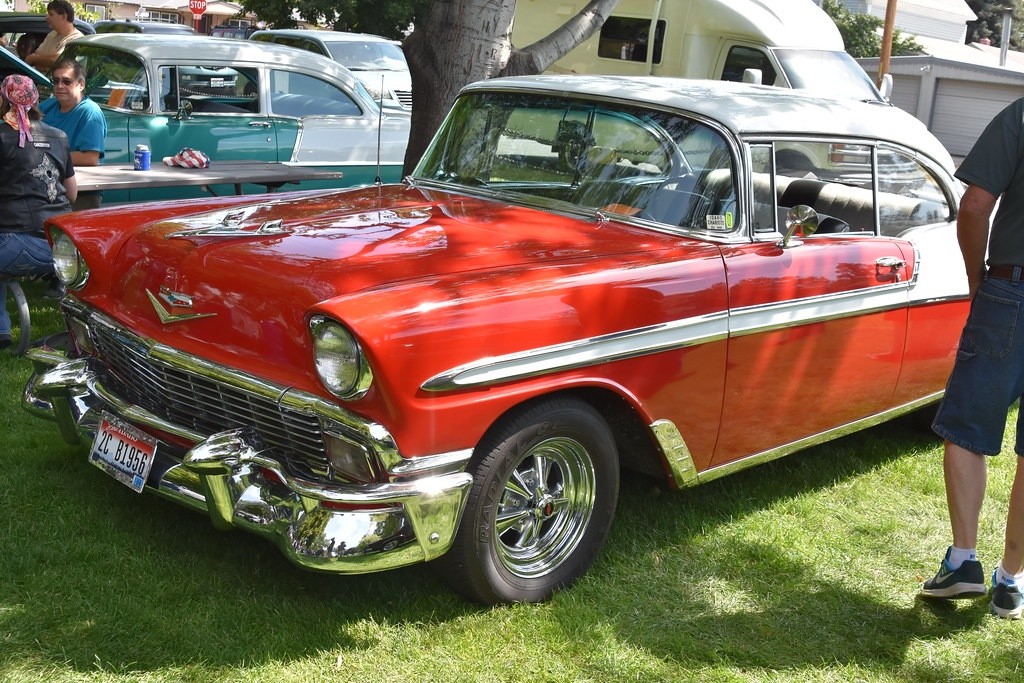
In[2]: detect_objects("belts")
[988,266,1024,281]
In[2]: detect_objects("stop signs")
[186,0,207,15]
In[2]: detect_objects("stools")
[0,271,39,357]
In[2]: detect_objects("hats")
[162,147,212,168]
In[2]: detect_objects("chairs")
[569,180,715,229]
[161,96,250,113]
[716,199,850,238]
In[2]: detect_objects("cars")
[0,11,413,205]
[20,72,998,608]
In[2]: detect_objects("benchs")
[674,168,945,232]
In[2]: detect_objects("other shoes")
[43,274,68,300]
[0,333,12,345]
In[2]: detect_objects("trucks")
[498,1,926,196]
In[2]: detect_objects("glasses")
[50,77,74,86]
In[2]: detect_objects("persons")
[11,0,89,102]
[33,58,106,167]
[920,99,1024,619]
[0,75,78,345]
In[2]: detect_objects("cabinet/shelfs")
[250,93,362,117]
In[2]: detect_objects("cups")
[134,150,151,170]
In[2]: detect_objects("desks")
[71,161,344,197]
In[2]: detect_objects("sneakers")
[921,546,987,598]
[991,566,1024,619]
